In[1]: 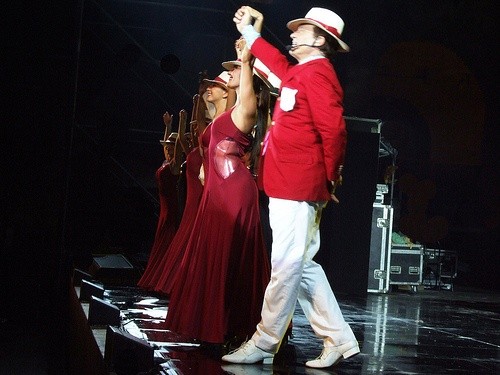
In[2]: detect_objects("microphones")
[285,44,315,51]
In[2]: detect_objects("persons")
[223,7,361,368]
[139,6,294,346]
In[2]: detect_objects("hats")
[287,7,350,52]
[189,110,213,124]
[160,132,180,146]
[202,70,230,90]
[221,54,271,90]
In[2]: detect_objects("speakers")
[311,121,381,298]
[72,269,91,287]
[104,325,154,375]
[87,294,121,328]
[85,252,134,285]
[78,279,105,303]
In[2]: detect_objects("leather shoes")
[222,339,274,365]
[305,339,360,368]
